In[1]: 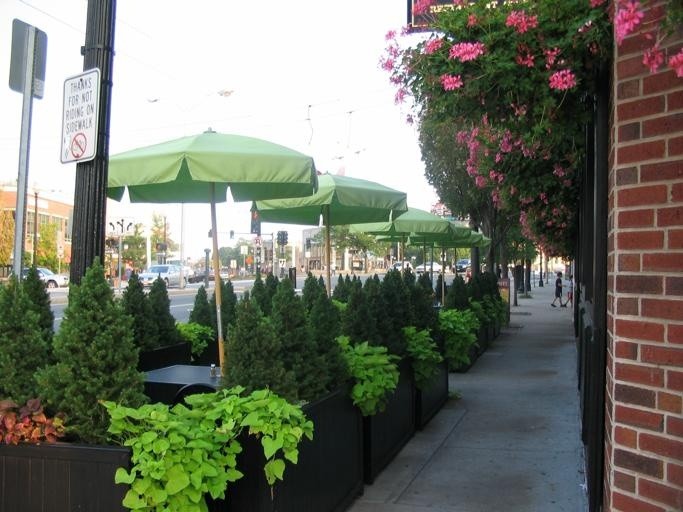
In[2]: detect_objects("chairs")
[168,381,220,409]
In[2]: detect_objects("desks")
[142,361,226,398]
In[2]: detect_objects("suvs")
[455,258,471,271]
[6,267,69,289]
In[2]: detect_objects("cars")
[136,264,187,288]
[387,260,413,275]
[176,266,235,284]
[415,262,442,273]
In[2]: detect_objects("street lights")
[31,181,40,267]
[107,219,133,299]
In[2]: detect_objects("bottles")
[210,364,216,379]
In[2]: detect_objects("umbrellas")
[107,127,318,379]
[349,206,492,307]
[250,171,408,299]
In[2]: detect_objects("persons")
[564,275,573,307]
[551,272,564,307]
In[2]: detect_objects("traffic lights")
[104,236,117,249]
[305,238,311,250]
[155,242,166,252]
[276,231,282,245]
[282,231,287,245]
